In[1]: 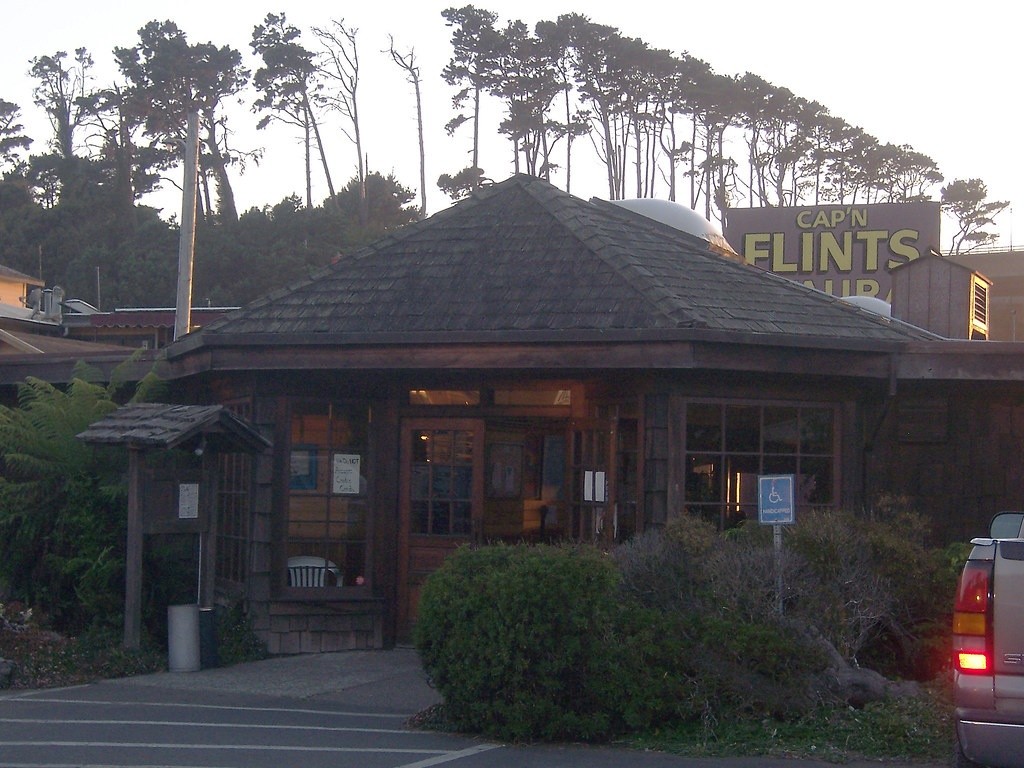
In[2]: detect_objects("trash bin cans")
[166,604,219,673]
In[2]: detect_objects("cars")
[950,513,1024,768]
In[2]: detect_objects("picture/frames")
[286,441,319,491]
